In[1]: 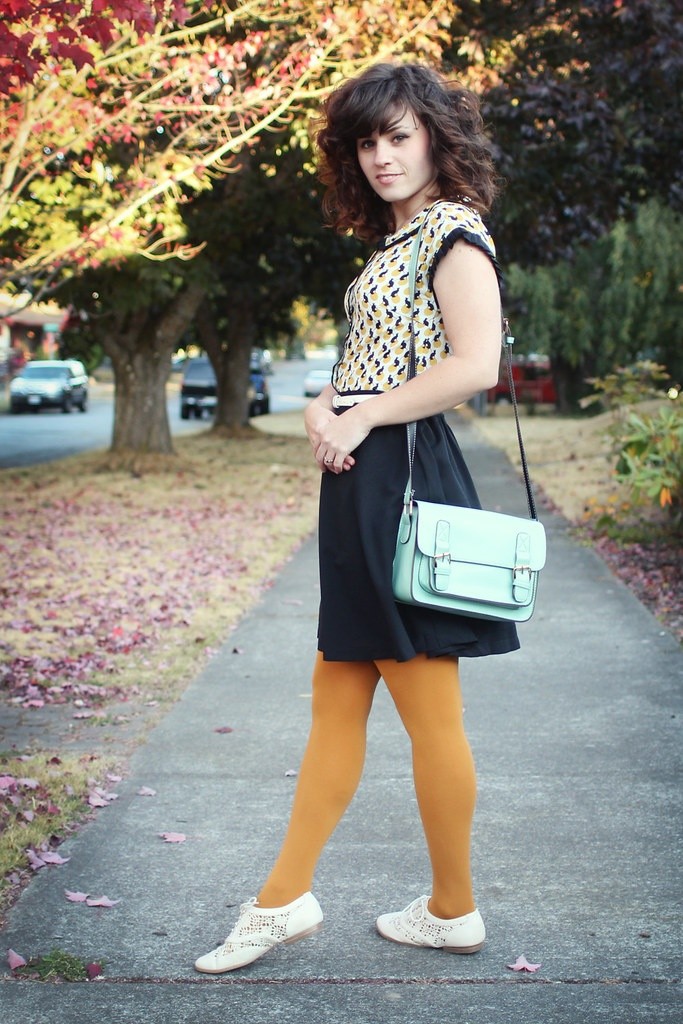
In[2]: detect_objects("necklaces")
[384,185,439,238]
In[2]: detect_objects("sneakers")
[195,891,323,974]
[376,894,486,953]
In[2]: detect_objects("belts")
[332,395,380,409]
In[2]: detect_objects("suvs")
[9,360,90,413]
[181,356,270,420]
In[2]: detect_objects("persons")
[194,65,521,973]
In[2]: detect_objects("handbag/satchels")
[389,500,546,622]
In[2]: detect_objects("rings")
[324,457,333,463]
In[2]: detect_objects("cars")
[307,370,335,396]
[488,354,559,407]
